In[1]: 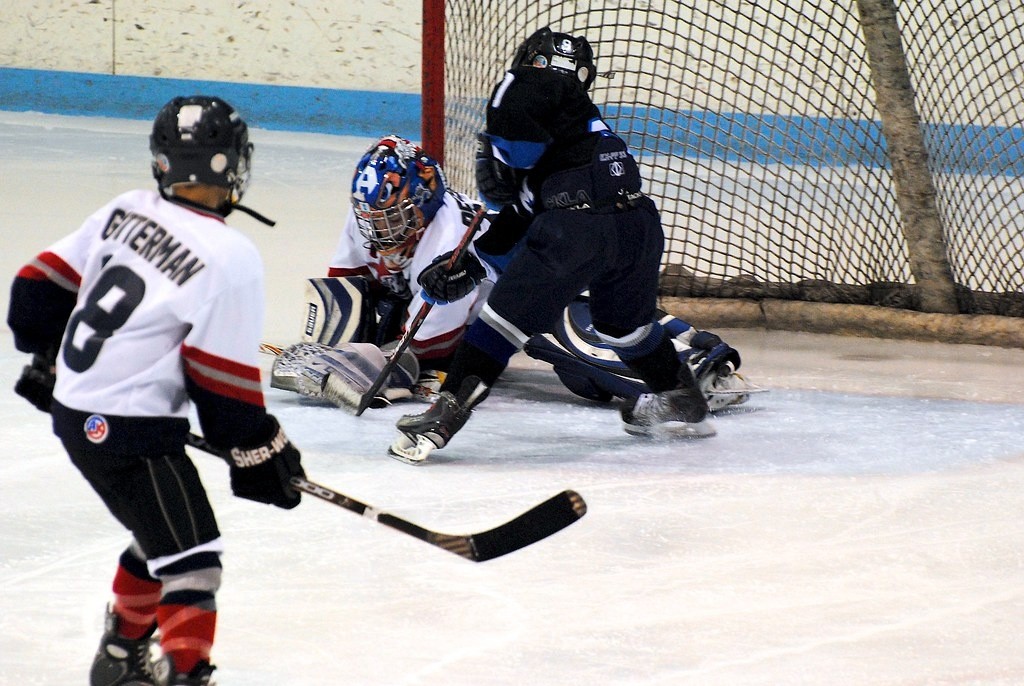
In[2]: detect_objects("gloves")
[477,133,520,205]
[14,348,57,412]
[224,414,307,509]
[418,248,485,304]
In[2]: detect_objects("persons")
[393,24,713,453]
[271,132,749,412]
[6,94,305,686]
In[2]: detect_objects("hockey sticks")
[183,432,589,562]
[322,203,490,420]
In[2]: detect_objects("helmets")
[512,25,599,89]
[352,133,447,229]
[151,95,248,189]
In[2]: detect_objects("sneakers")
[90,603,216,686]
[389,374,490,466]
[655,314,770,410]
[621,367,717,438]
